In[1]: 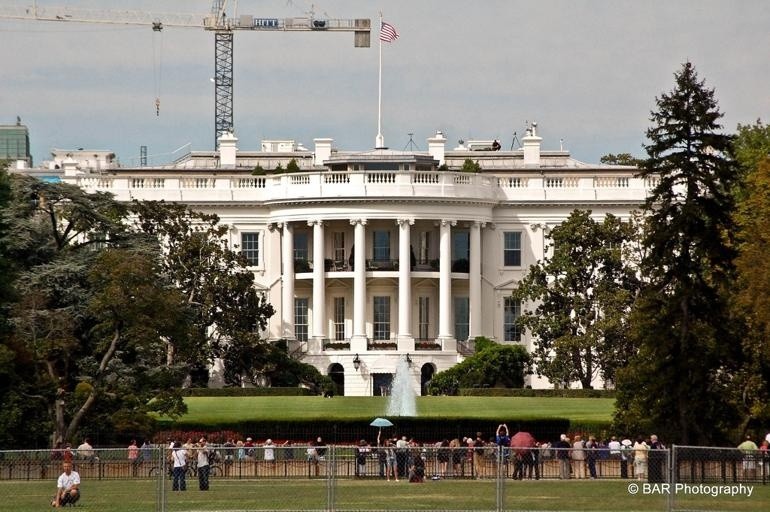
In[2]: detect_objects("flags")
[380,21,399,42]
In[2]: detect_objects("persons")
[49,461,81,508]
[52,423,769,493]
[491,139,502,151]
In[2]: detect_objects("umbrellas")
[370,418,395,431]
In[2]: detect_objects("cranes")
[0,1,371,154]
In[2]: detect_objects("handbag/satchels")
[181,464,188,473]
[357,452,366,465]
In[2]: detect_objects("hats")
[267,439,272,444]
[358,440,367,446]
[64,443,72,447]
[247,437,252,441]
[622,439,632,446]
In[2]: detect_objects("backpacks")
[473,439,484,456]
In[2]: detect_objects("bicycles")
[148,460,224,478]
